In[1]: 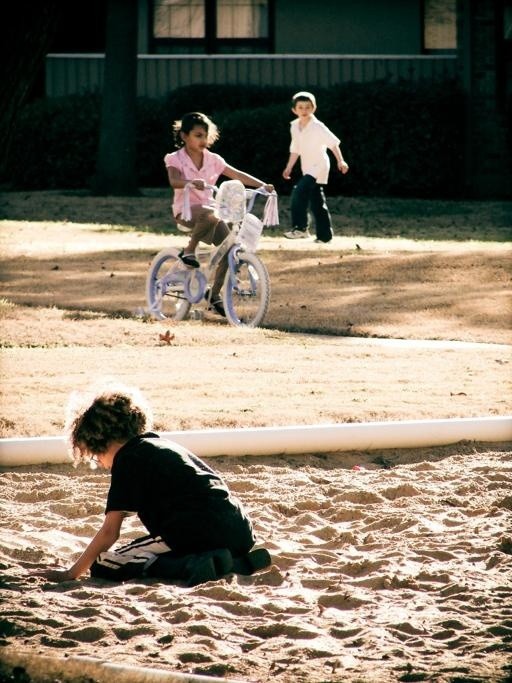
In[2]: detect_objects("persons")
[29,393,270,584]
[164,114,273,319]
[281,92,347,247]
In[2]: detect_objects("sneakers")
[233,549,271,574]
[187,549,233,587]
[284,226,311,239]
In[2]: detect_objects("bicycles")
[146,181,277,328]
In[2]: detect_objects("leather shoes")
[205,289,226,318]
[178,248,200,269]
